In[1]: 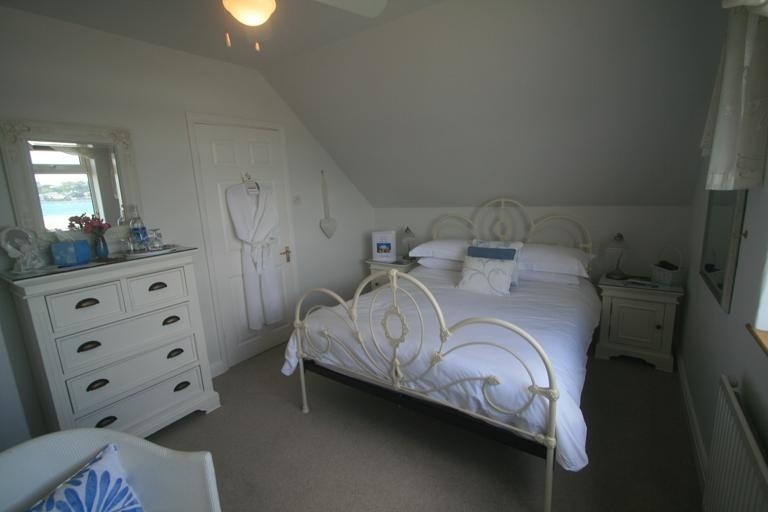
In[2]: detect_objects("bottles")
[129,203,148,252]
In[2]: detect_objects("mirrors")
[1,119,144,265]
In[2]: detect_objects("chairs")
[1,427,220,512]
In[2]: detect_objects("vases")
[94,237,109,258]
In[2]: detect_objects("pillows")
[29,442,142,511]
[409,240,597,295]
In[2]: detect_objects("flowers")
[84,210,111,236]
[67,212,91,231]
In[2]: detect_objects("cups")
[147,228,163,251]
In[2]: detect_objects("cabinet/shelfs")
[0,245,222,439]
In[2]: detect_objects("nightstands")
[364,260,411,289]
[597,275,685,373]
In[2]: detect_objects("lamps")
[399,226,417,260]
[606,231,626,279]
[222,0,276,51]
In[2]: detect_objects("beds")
[281,198,592,512]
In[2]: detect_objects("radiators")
[701,374,768,512]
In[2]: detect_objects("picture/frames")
[695,190,747,314]
[371,230,396,264]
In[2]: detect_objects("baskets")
[647,247,684,286]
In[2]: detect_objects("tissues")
[50,228,91,267]
